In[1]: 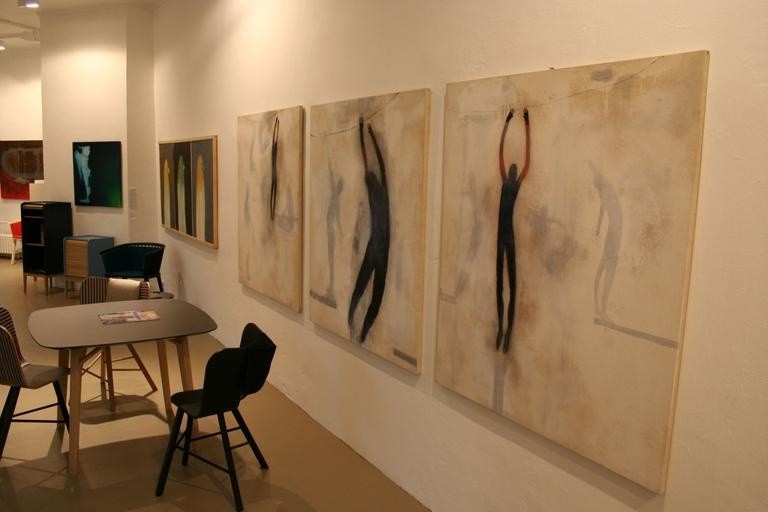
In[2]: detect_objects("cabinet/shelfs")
[20,201,73,295]
[63,236,115,296]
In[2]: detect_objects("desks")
[28,299,217,476]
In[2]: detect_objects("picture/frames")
[158,135,219,249]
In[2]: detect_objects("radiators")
[0,234,13,254]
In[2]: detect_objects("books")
[97,310,160,326]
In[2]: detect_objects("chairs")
[156,322,277,512]
[10,221,22,264]
[0,307,69,464]
[101,242,165,292]
[67,276,158,411]
